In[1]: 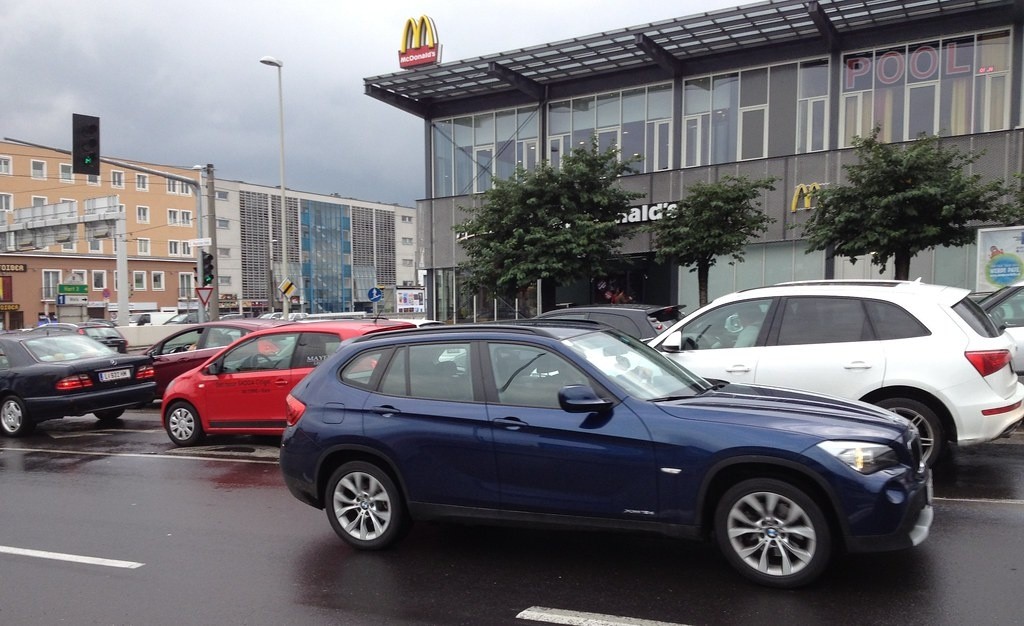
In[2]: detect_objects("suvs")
[277,318,934,590]
[621,277,1024,473]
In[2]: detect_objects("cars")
[976,278,1024,376]
[0,317,128,355]
[0,332,157,439]
[125,319,303,410]
[160,320,417,447]
[128,303,686,385]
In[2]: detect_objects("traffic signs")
[57,294,89,306]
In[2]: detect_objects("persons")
[610,287,632,304]
[188,328,220,351]
[711,303,765,347]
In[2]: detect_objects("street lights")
[192,163,220,322]
[258,55,287,319]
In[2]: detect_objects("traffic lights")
[71,113,100,175]
[201,250,214,287]
[193,266,199,283]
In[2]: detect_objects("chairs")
[434,362,467,399]
[218,334,233,346]
